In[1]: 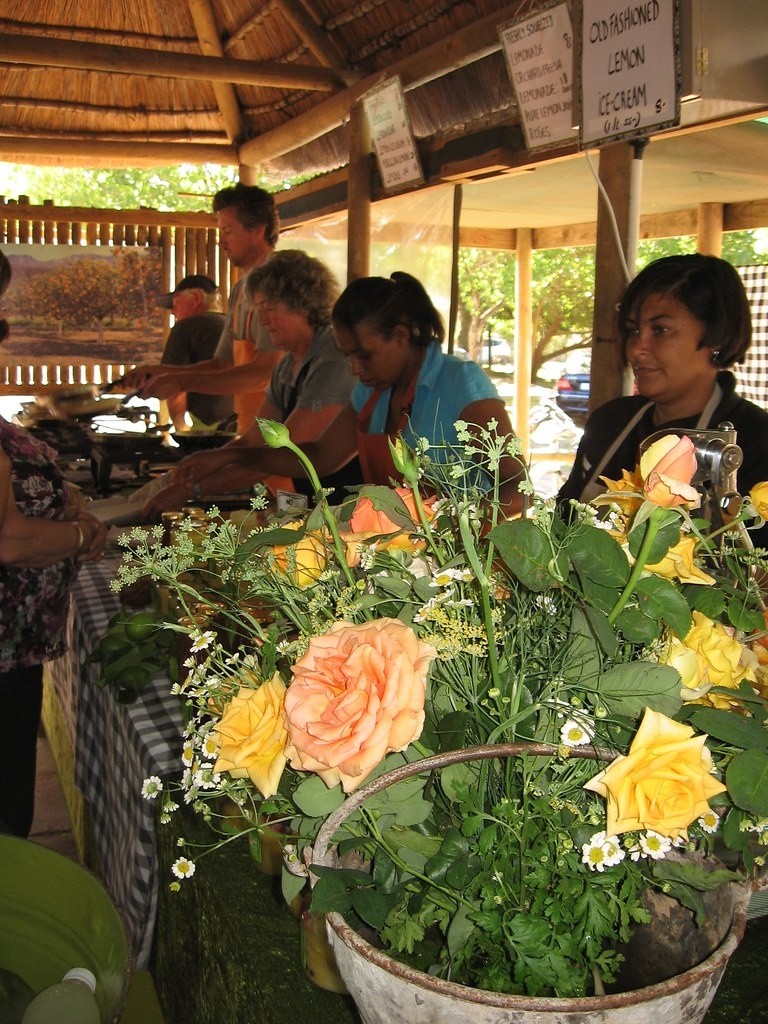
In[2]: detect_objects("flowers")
[110,399,768,997]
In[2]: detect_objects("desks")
[42,545,361,1024]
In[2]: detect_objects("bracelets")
[72,521,83,552]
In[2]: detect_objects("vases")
[308,742,750,1024]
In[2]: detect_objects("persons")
[555,255,768,563]
[0,248,107,838]
[172,270,531,538]
[155,273,235,431]
[128,249,364,516]
[120,183,295,496]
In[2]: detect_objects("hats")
[155,275,217,309]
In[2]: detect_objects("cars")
[478,338,513,374]
[553,355,639,418]
